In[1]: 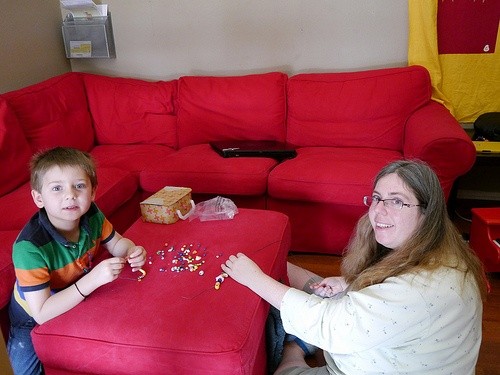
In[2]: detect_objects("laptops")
[210,141,298,157]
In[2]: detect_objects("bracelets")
[74,283,86,298]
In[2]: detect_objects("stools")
[470,206,500,272]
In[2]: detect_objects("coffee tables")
[30,207,292,375]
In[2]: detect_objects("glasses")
[363,194,422,213]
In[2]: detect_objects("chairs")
[446,112,500,216]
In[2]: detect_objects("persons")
[7,147,147,375]
[221,159,490,375]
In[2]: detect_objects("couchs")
[0,64,477,346]
